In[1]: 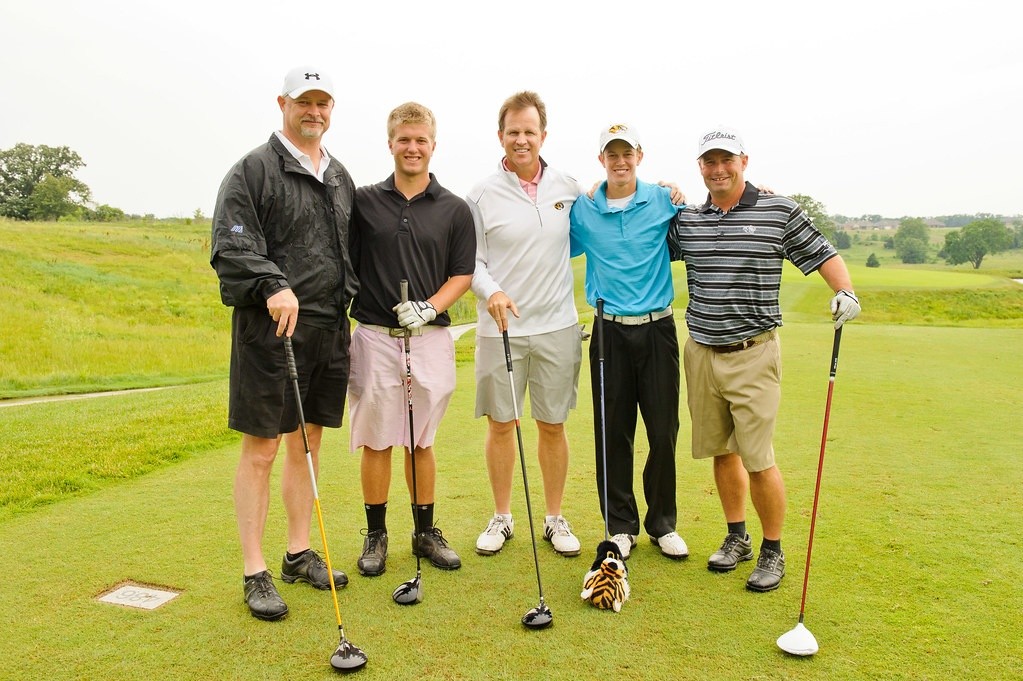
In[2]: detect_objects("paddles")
[504,328,556,630]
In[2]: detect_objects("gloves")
[830,290,861,329]
[392,300,437,330]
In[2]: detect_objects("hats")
[696,129,745,159]
[599,122,642,153]
[282,67,335,101]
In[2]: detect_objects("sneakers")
[476,515,514,555]
[543,516,582,556]
[609,533,637,559]
[650,531,689,558]
[411,518,461,569]
[281,549,348,590]
[243,571,289,619]
[357,528,388,575]
[746,547,785,591]
[708,533,754,570]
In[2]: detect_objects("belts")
[594,306,673,325]
[695,340,754,353]
[358,322,442,336]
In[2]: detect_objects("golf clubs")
[775,325,843,657]
[282,330,367,672]
[392,278,424,606]
[596,298,609,539]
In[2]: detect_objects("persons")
[464,92,687,556]
[570,126,775,559]
[348,102,477,577]
[210,64,357,622]
[586,130,861,592]
